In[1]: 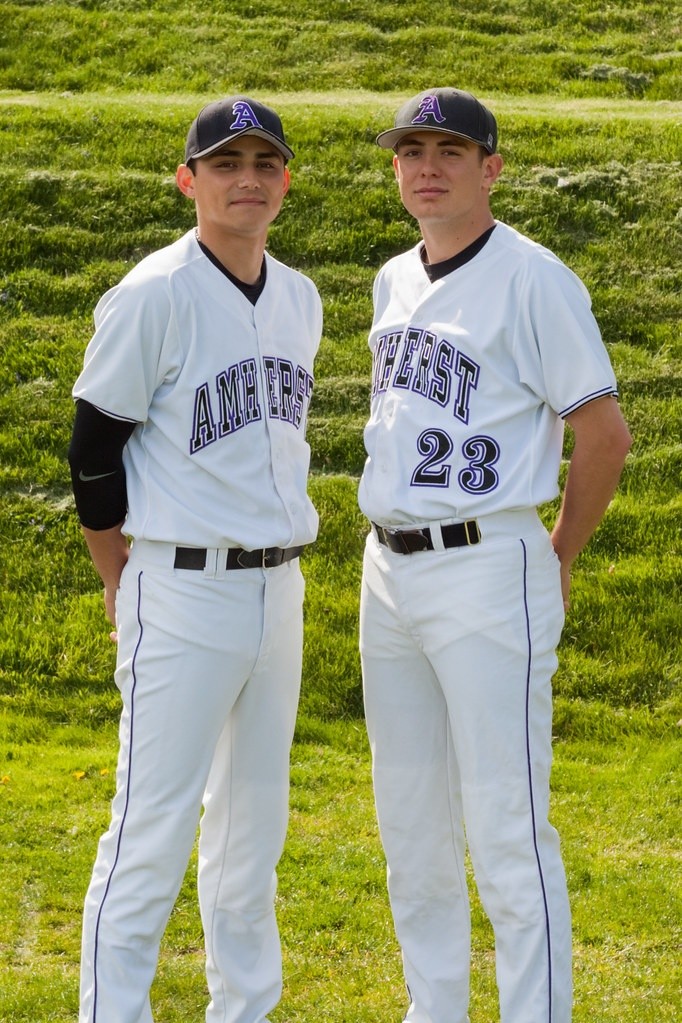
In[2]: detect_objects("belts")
[372,519,481,555]
[173,546,305,570]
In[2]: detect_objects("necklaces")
[195,233,200,241]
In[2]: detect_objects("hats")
[376,88,497,155]
[186,95,295,169]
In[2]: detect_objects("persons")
[358,86,633,1023]
[69,96,324,1023]
